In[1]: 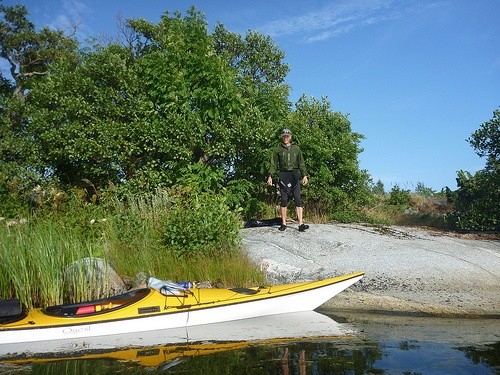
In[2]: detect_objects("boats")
[1,271,367,361]
[0,312,356,372]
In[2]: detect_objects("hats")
[280,129,292,137]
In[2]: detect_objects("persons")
[266,129,309,233]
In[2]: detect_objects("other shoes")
[298,223,309,231]
[279,223,287,231]
